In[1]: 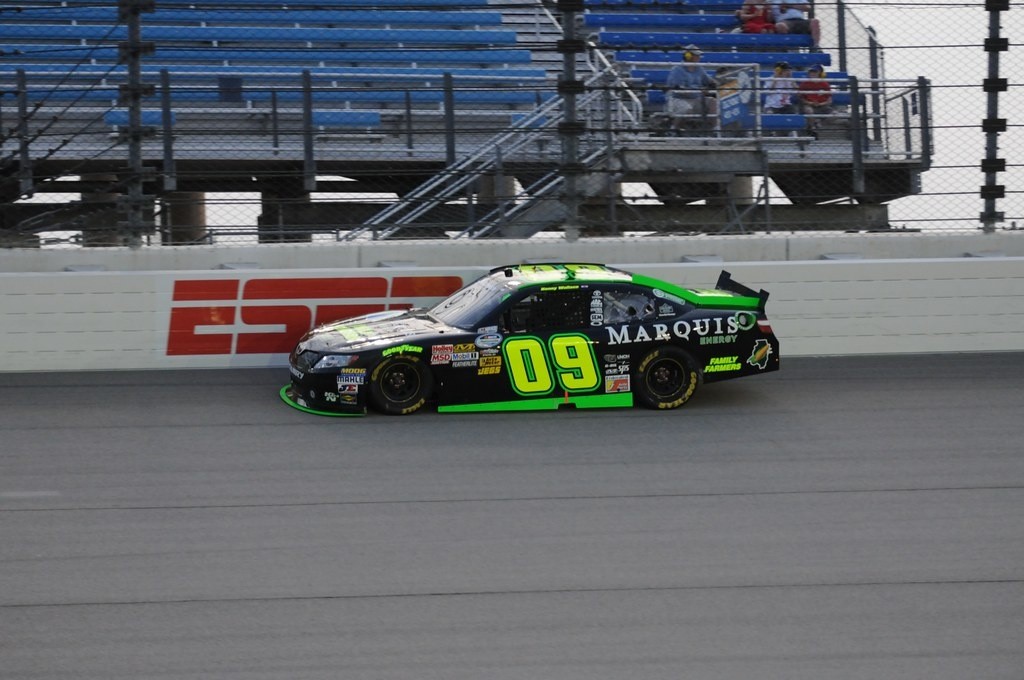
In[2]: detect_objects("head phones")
[775,62,788,74]
[808,64,826,78]
[685,51,692,60]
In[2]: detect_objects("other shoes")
[808,131,818,140]
[810,46,823,53]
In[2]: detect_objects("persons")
[735,0,823,52]
[799,64,851,141]
[763,63,798,137]
[666,46,754,137]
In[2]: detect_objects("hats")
[808,65,820,73]
[777,61,792,69]
[684,44,704,55]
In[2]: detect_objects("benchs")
[0,0,864,139]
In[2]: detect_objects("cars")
[276,260,781,419]
